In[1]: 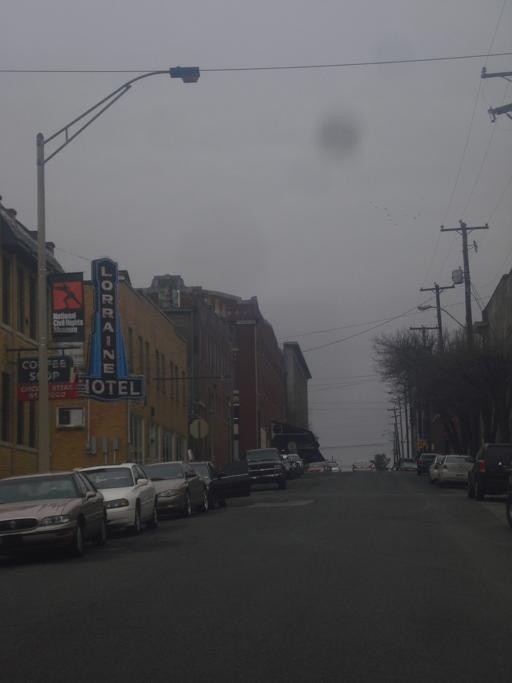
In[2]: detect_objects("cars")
[415,441,512,533]
[306,458,417,472]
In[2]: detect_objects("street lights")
[36,64,201,475]
[415,302,466,329]
